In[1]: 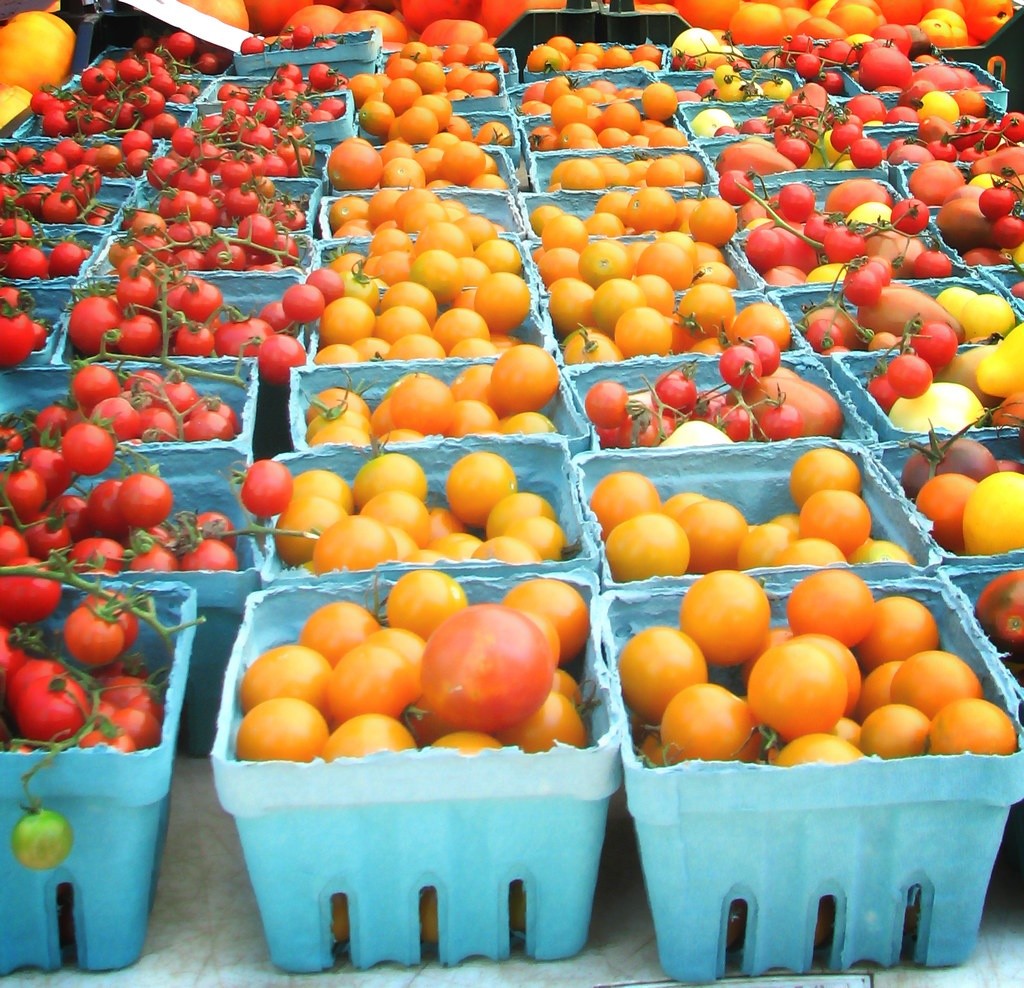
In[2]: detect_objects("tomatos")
[0,0,1024,944]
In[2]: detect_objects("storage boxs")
[1,0,1024,984]
